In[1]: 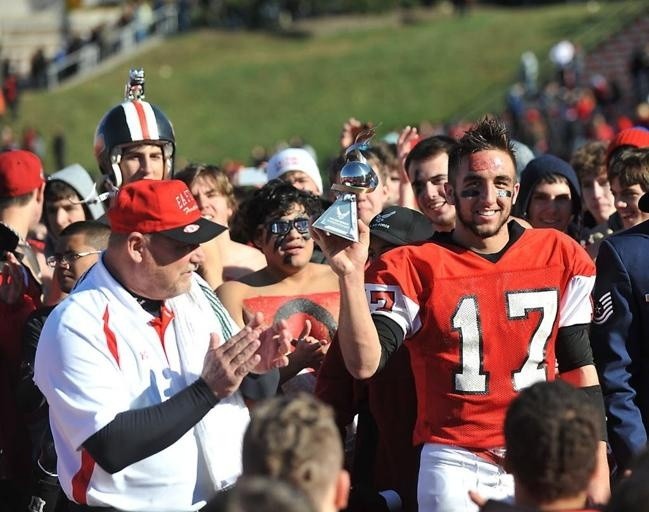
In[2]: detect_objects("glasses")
[41,250,101,265]
[265,216,311,236]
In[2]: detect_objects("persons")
[469,381,606,512]
[34,179,291,512]
[267,147,322,203]
[18,220,112,512]
[568,142,616,225]
[174,163,267,282]
[0,150,45,512]
[405,135,456,232]
[43,164,106,239]
[215,179,340,393]
[502,34,649,153]
[588,188,649,472]
[309,118,610,512]
[312,207,436,512]
[329,149,390,232]
[243,395,350,512]
[518,155,582,247]
[609,148,649,230]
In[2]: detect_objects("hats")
[266,148,322,195]
[109,178,230,248]
[0,150,46,198]
[604,127,649,154]
[367,204,436,245]
